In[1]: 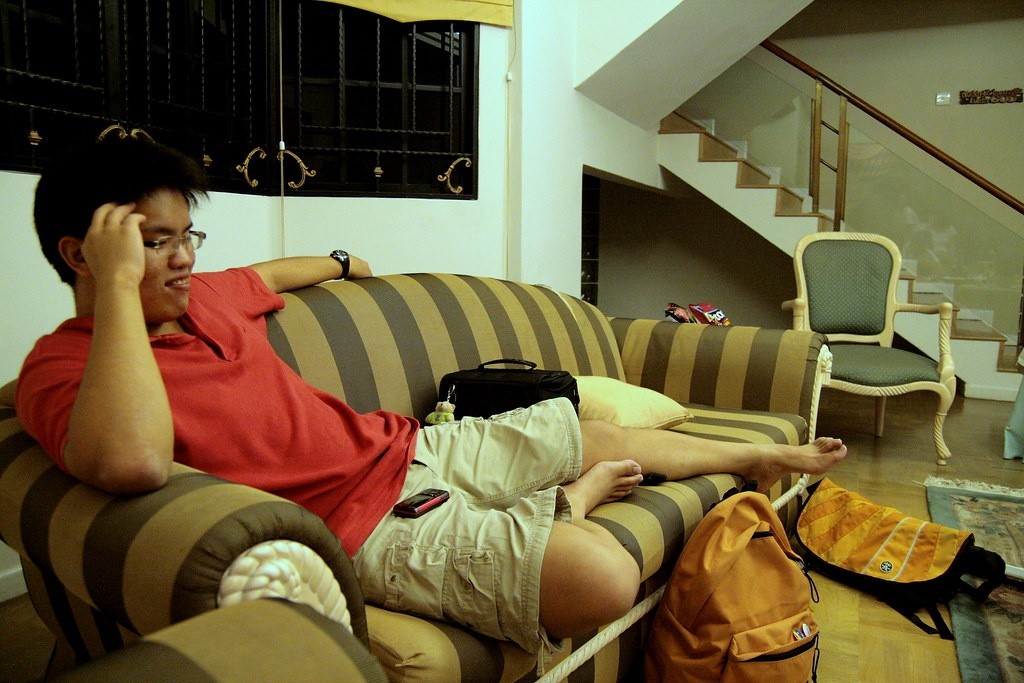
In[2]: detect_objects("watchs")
[331,250,350,278]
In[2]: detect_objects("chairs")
[780,232,958,465]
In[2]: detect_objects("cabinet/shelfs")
[0,0,514,392]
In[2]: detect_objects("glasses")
[144,230,206,255]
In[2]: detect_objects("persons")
[16,140,848,656]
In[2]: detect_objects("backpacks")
[645,488,822,683]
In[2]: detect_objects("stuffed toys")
[426,401,456,425]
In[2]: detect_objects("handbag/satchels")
[795,473,1006,609]
[437,357,580,421]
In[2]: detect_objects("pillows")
[572,373,693,437]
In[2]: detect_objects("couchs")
[0,270,833,683]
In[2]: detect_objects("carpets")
[917,476,1024,683]
